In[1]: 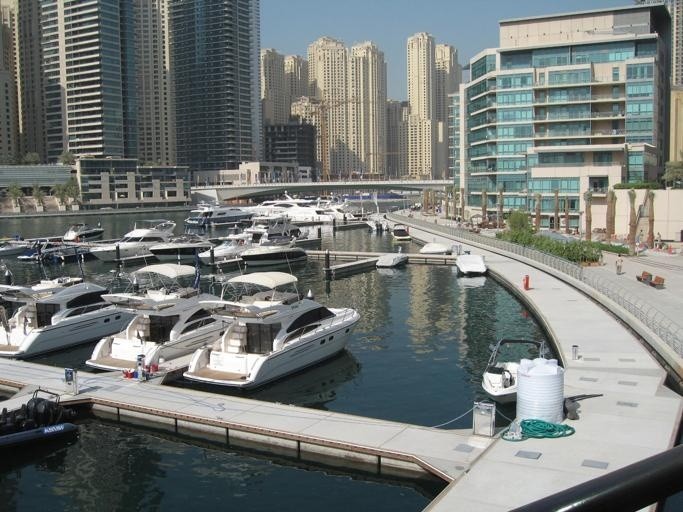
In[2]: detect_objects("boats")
[84,253,244,372]
[1,386,80,471]
[419,241,452,256]
[456,252,487,275]
[391,223,410,241]
[0,221,176,260]
[191,190,375,227]
[0,264,137,361]
[376,252,408,269]
[148,218,308,267]
[481,337,562,405]
[181,268,361,387]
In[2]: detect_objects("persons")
[614,254,623,276]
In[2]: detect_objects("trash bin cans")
[65,369,74,382]
[524,275,529,290]
[572,345,578,360]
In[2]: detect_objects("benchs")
[636,271,665,289]
